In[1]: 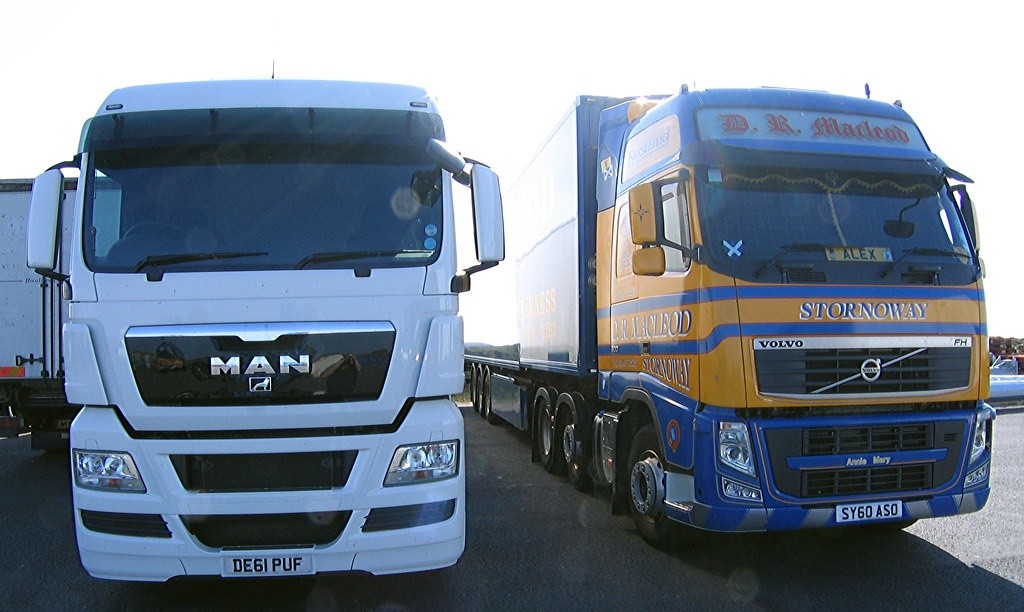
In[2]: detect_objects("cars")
[983,355,1024,409]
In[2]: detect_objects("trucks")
[27,79,505,582]
[0,176,124,451]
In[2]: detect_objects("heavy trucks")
[457,83,996,547]
